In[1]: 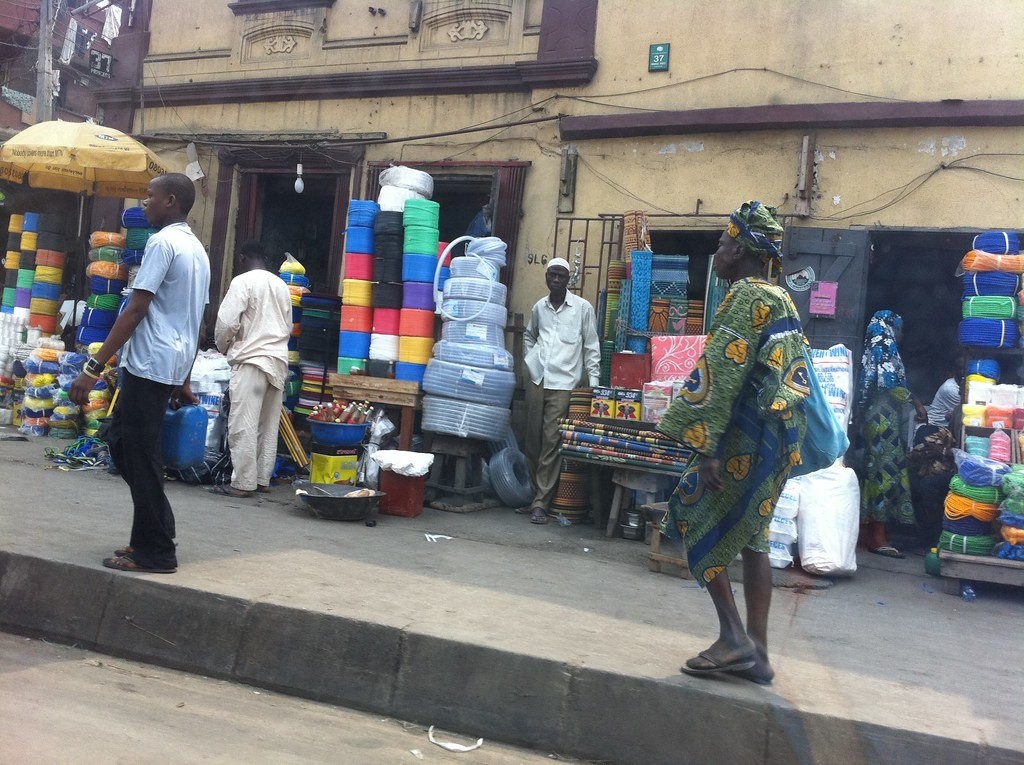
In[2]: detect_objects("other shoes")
[914,546,929,557]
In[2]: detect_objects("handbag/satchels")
[787,347,851,479]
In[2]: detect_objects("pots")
[297,484,387,520]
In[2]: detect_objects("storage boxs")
[337,357,368,376]
[615,389,642,422]
[642,382,673,424]
[610,353,651,389]
[310,443,362,456]
[395,362,427,392]
[309,452,358,487]
[589,388,616,419]
[365,359,395,378]
[1014,430,1024,464]
[284,396,299,410]
[623,527,642,540]
[285,380,302,397]
[292,412,312,432]
[379,467,426,517]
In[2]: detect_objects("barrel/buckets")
[379,465,425,518]
[157,399,208,471]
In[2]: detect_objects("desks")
[328,374,423,452]
[561,455,683,477]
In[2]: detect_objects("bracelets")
[84,358,103,379]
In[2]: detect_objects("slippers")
[104,555,177,573]
[530,507,548,523]
[257,488,270,494]
[732,668,771,686]
[114,548,130,556]
[208,485,249,498]
[869,545,904,558]
[681,650,756,674]
[515,506,531,514]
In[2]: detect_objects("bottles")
[308,399,374,424]
[961,580,977,602]
[358,359,369,376]
[924,548,938,574]
[387,361,395,378]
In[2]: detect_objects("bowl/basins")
[624,509,642,527]
[306,416,373,447]
[621,524,644,540]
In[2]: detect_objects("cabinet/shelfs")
[937,345,1024,596]
[961,424,1017,465]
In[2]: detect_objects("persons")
[927,357,966,427]
[857,311,927,556]
[67,174,212,574]
[517,257,605,523]
[658,204,839,688]
[205,244,292,498]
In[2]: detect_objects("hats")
[547,258,570,271]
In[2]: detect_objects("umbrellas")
[0,119,170,239]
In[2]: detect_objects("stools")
[421,436,492,507]
[604,467,672,545]
[641,501,693,580]
